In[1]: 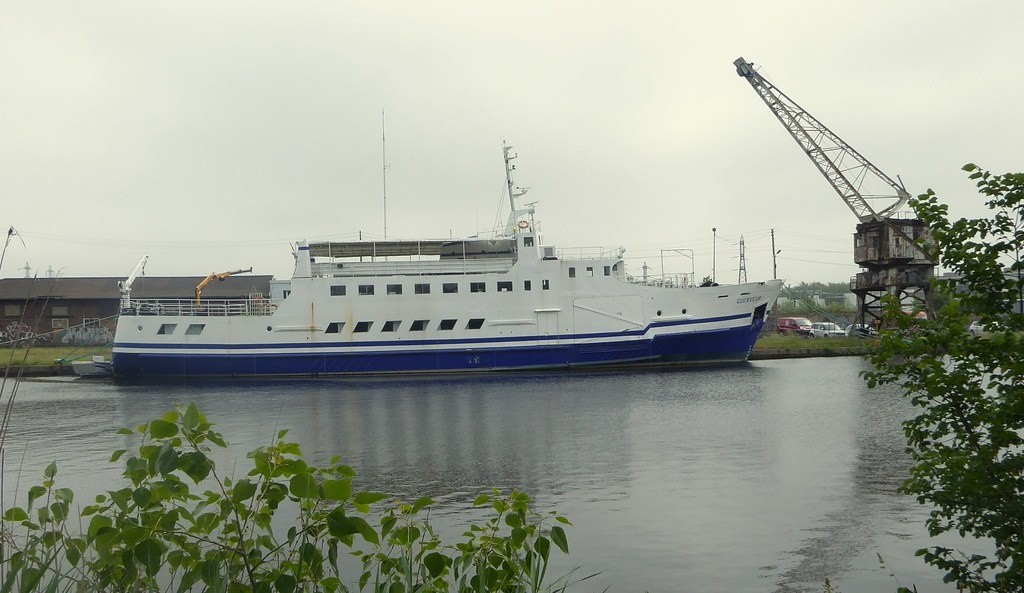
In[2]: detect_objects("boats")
[71,356,113,377]
[113,140,786,380]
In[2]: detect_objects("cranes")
[195,268,253,313]
[734,57,940,339]
[116,255,149,314]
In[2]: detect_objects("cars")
[845,323,879,338]
[969,320,1010,340]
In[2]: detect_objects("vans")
[777,317,812,336]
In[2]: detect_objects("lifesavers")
[518,220,529,229]
[255,297,266,307]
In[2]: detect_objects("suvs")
[809,321,845,338]
